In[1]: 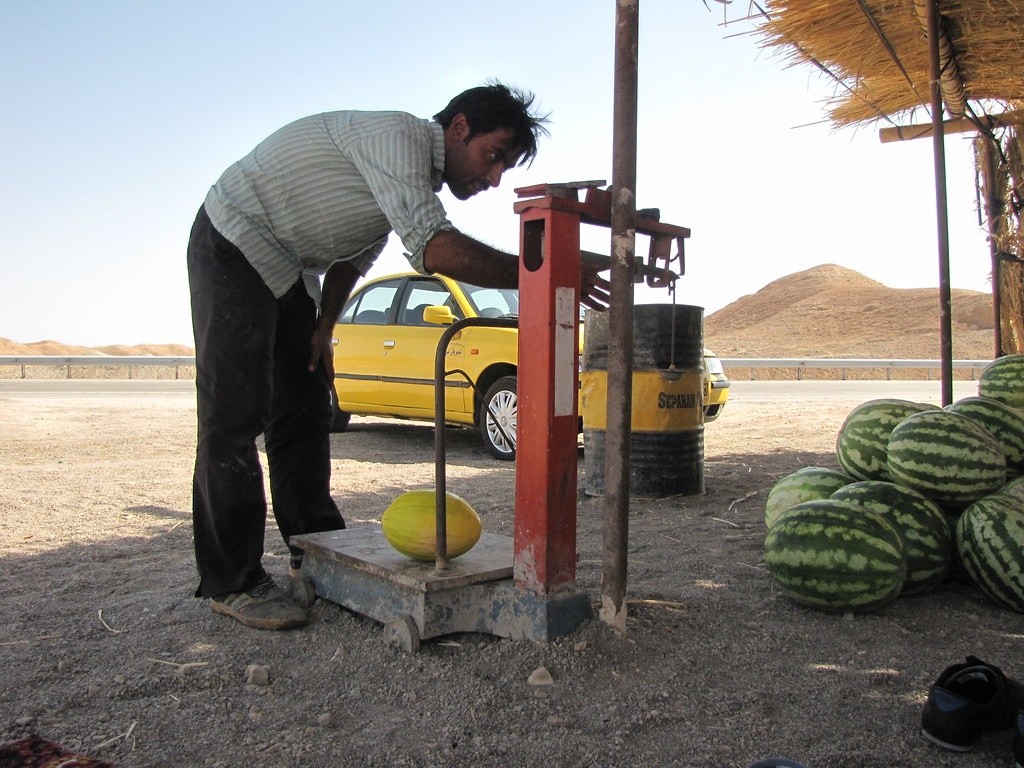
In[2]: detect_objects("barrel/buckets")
[582,304,706,499]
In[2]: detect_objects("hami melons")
[383,490,481,562]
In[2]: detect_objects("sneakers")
[210,573,308,629]
[289,565,301,578]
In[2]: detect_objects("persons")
[186,83,610,632]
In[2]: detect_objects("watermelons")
[766,356,1024,612]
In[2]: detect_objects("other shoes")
[920,654,1024,768]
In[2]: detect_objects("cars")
[329,271,731,461]
